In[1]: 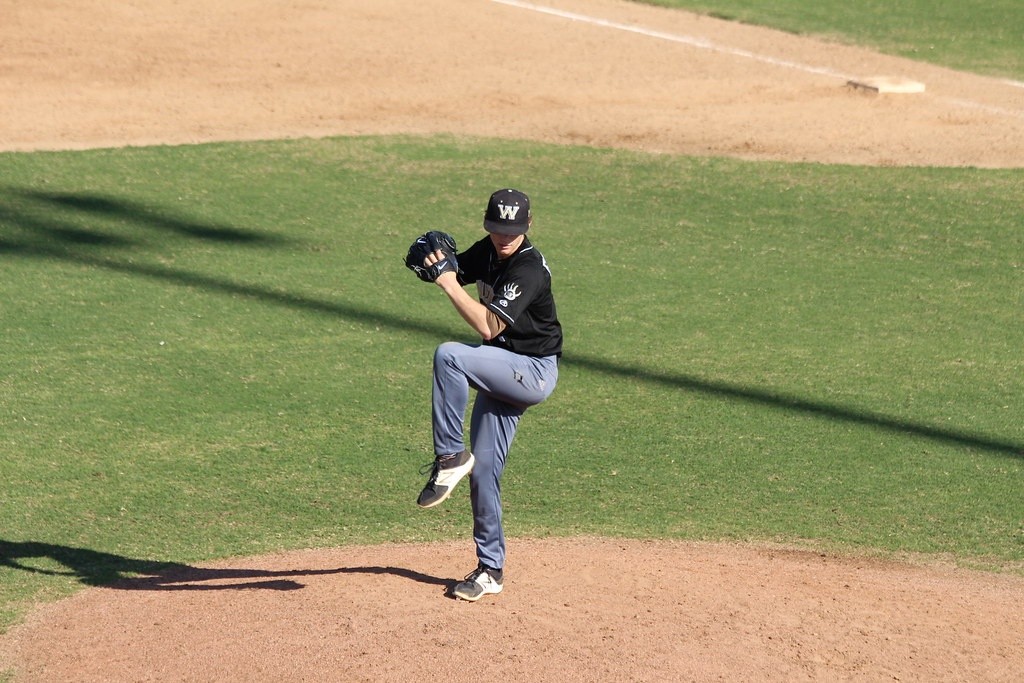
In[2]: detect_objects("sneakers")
[452,562,504,601]
[417,448,475,509]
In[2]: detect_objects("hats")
[484,188,530,235]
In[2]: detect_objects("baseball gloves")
[405,230,459,284]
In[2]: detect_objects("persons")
[406,189,563,603]
